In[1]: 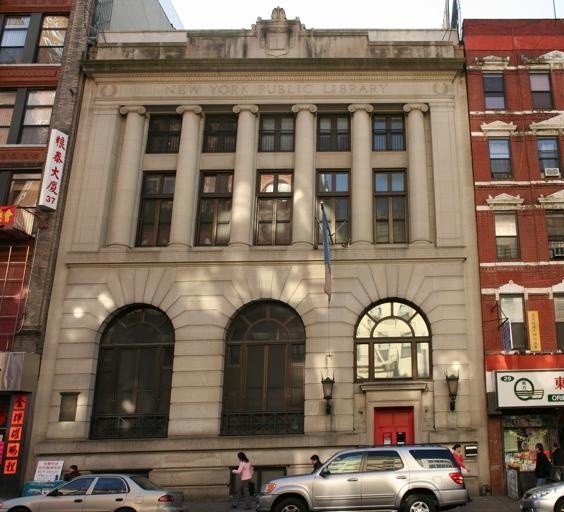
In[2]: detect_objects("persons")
[451,444,472,504]
[231,452,256,511]
[310,455,326,475]
[550,443,561,465]
[534,443,552,488]
[67,465,81,484]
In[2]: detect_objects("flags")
[321,207,333,306]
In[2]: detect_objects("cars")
[518,481,563,512]
[0,472,185,511]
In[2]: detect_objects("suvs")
[256,441,467,511]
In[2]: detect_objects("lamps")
[320,375,335,415]
[444,367,460,413]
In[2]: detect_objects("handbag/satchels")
[460,466,467,476]
[242,482,255,496]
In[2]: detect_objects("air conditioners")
[553,246,564,257]
[543,166,559,179]
